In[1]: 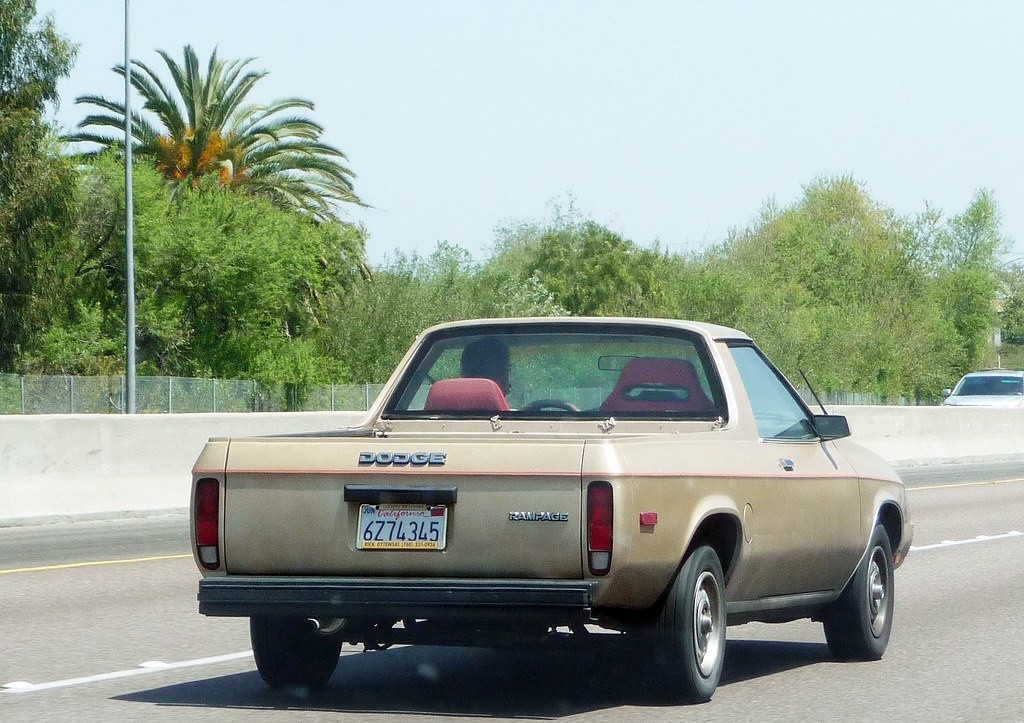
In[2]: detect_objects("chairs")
[598,358,714,412]
[423,378,510,411]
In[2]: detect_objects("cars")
[938,365,1024,408]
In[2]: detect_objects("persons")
[461,338,510,399]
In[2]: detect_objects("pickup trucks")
[182,312,916,701]
